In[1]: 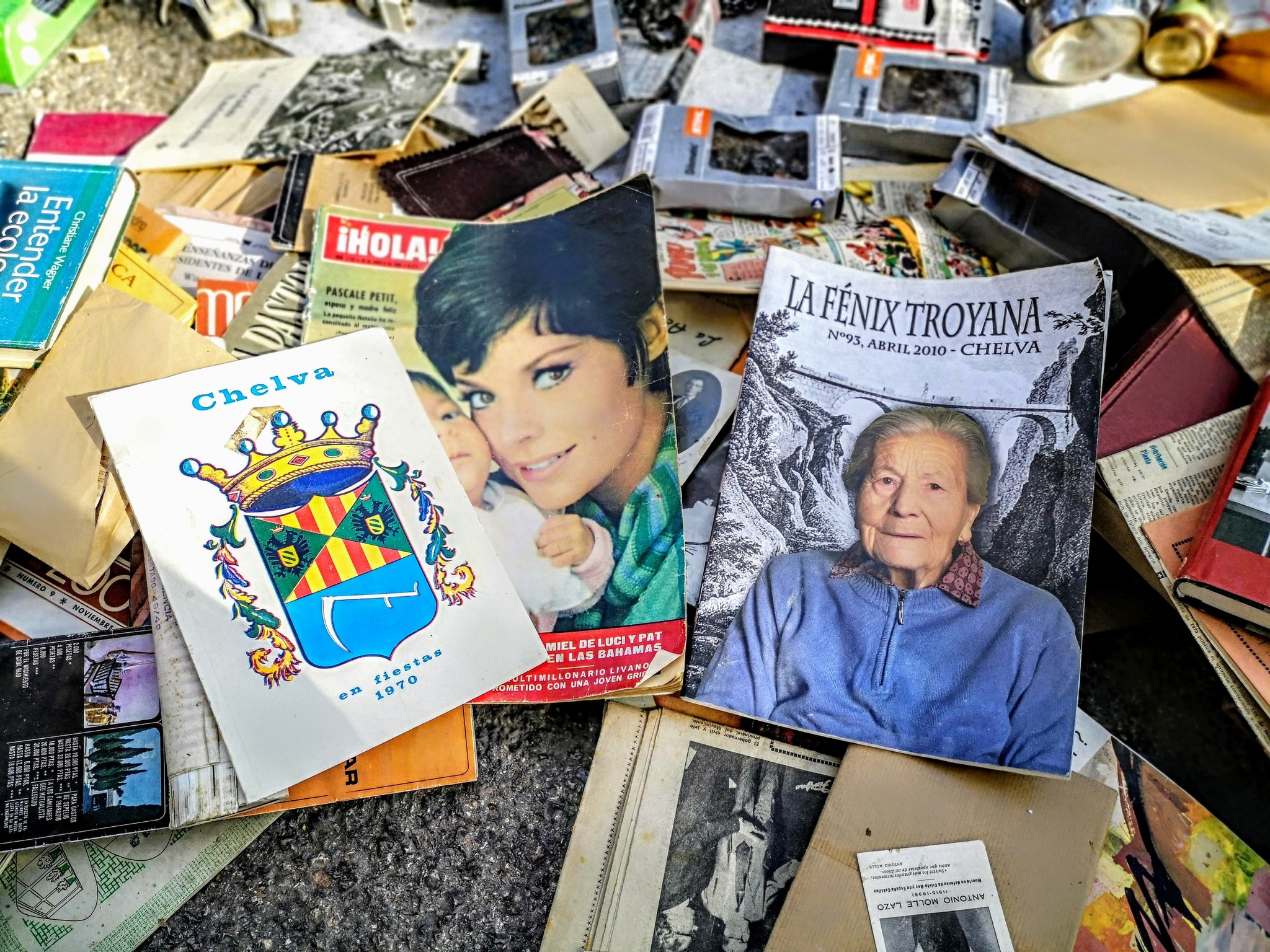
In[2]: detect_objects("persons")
[696,402,1081,779]
[701,756,799,952]
[405,368,615,632]
[412,181,687,631]
[653,744,762,951]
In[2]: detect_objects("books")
[0,49,1269,952]
